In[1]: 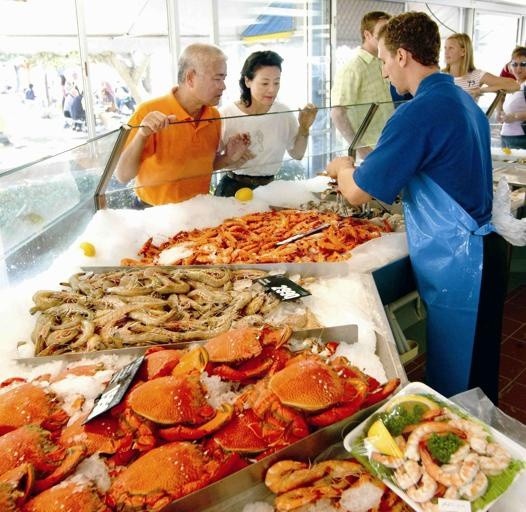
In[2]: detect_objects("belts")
[226,171,272,186]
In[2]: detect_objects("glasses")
[511,62,526,67]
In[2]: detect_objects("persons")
[490,44,526,148]
[24,83,35,100]
[439,31,521,105]
[500,45,523,81]
[113,42,253,212]
[69,85,86,132]
[323,9,511,411]
[214,49,320,199]
[327,8,397,163]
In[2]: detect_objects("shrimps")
[265,456,414,512]
[29,269,324,357]
[390,416,513,511]
[138,208,395,269]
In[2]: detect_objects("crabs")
[1,322,401,511]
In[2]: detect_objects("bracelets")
[514,112,518,120]
[298,129,310,137]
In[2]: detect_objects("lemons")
[79,241,97,258]
[235,188,253,202]
[368,420,404,459]
[388,393,441,419]
